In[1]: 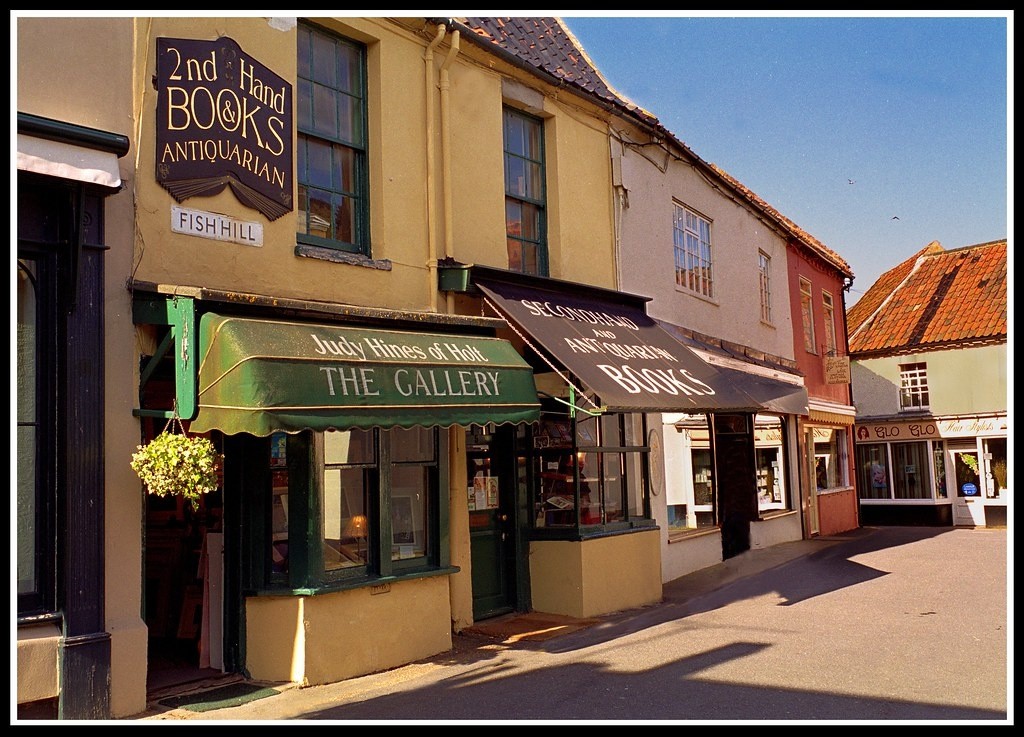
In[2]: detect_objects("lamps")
[341,514,367,560]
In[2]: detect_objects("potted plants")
[129,434,225,513]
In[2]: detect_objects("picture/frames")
[391,496,416,546]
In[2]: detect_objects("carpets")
[463,609,605,641]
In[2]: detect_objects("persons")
[181,502,209,584]
[568,465,592,523]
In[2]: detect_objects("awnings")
[185,313,542,437]
[479,282,768,425]
